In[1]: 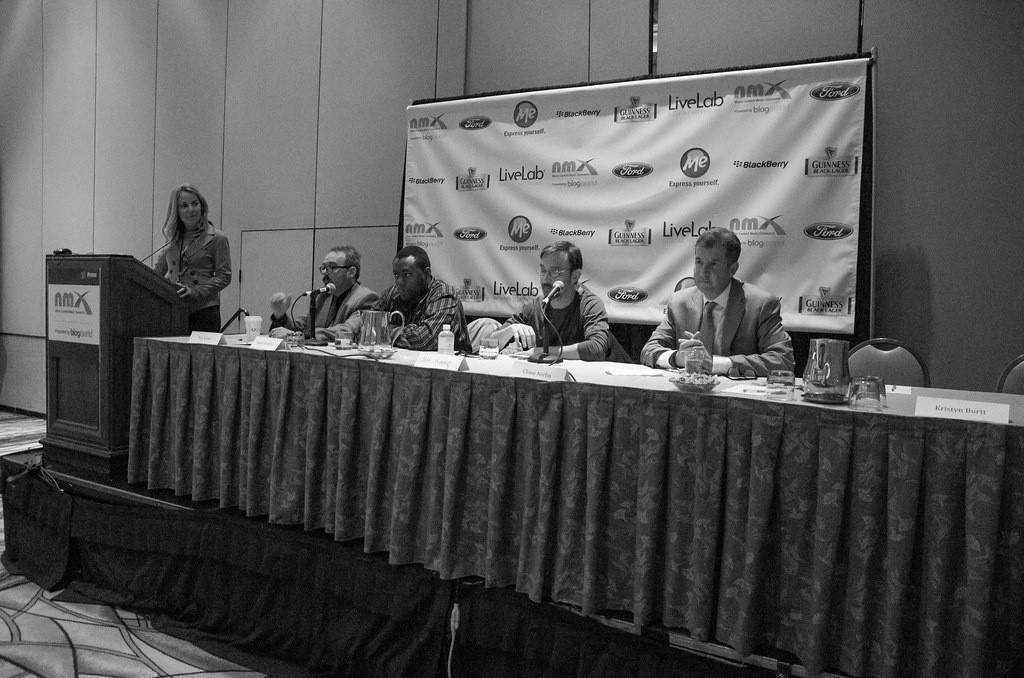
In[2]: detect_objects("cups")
[244,315,262,344]
[766,370,795,401]
[335,331,353,350]
[866,376,887,408]
[685,348,705,374]
[849,378,879,411]
[284,332,305,351]
[479,339,499,359]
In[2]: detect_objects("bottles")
[438,325,454,357]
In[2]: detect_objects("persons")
[153,183,232,336]
[640,226,796,377]
[268,246,380,345]
[353,246,472,355]
[486,240,634,365]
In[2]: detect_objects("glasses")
[535,265,572,277]
[721,367,757,380]
[319,265,349,274]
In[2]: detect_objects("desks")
[129,332,1024,678]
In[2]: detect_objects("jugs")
[804,339,850,403]
[358,310,404,351]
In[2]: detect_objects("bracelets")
[668,351,681,367]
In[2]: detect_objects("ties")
[326,298,340,329]
[701,301,717,358]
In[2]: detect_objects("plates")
[669,377,720,392]
[360,347,398,359]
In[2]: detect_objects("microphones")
[140,232,177,262]
[541,280,565,305]
[302,283,336,296]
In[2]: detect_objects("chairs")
[847,338,930,387]
[466,318,502,352]
